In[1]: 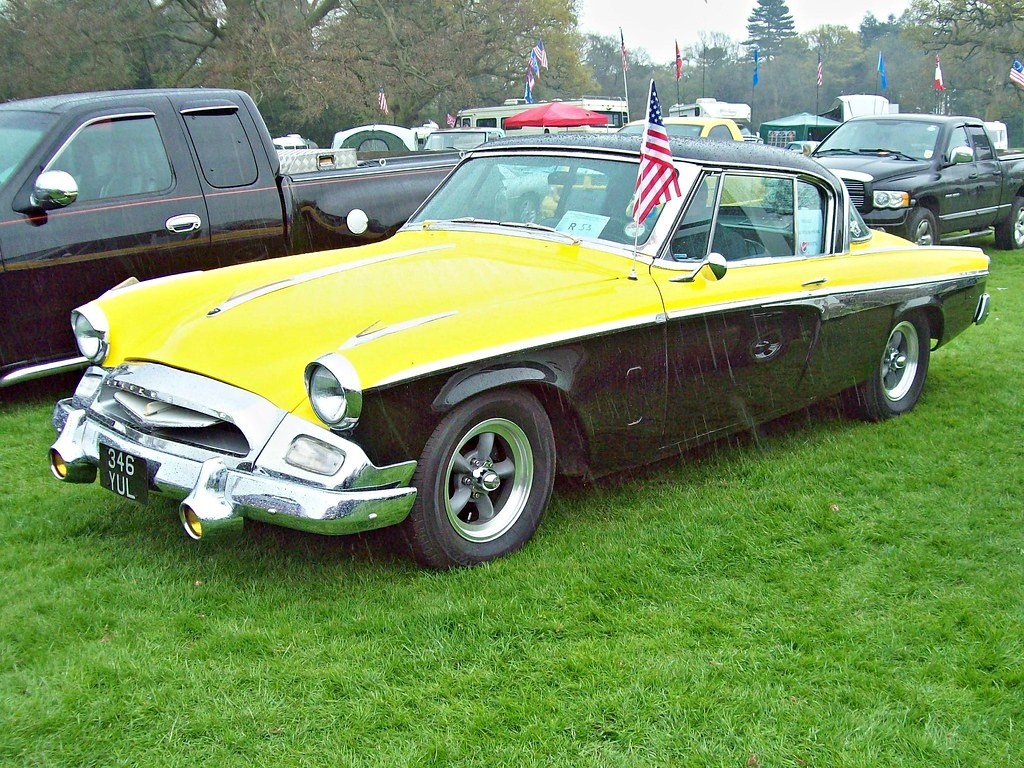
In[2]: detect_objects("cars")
[44,132,993,574]
[272,122,570,225]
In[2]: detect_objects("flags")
[626,82,681,222]
[935,56,944,91]
[878,52,887,89]
[533,42,548,69]
[621,31,627,72]
[529,52,539,78]
[816,46,823,88]
[676,42,682,79]
[447,115,455,128]
[378,86,388,116]
[753,49,758,85]
[524,71,535,104]
[1009,59,1024,90]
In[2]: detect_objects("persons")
[671,180,747,261]
[802,144,811,156]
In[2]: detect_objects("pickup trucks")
[775,113,1024,251]
[540,118,771,221]
[0,88,509,390]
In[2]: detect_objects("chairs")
[53,139,98,201]
[101,141,161,198]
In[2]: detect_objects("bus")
[453,95,629,136]
[668,98,752,123]
